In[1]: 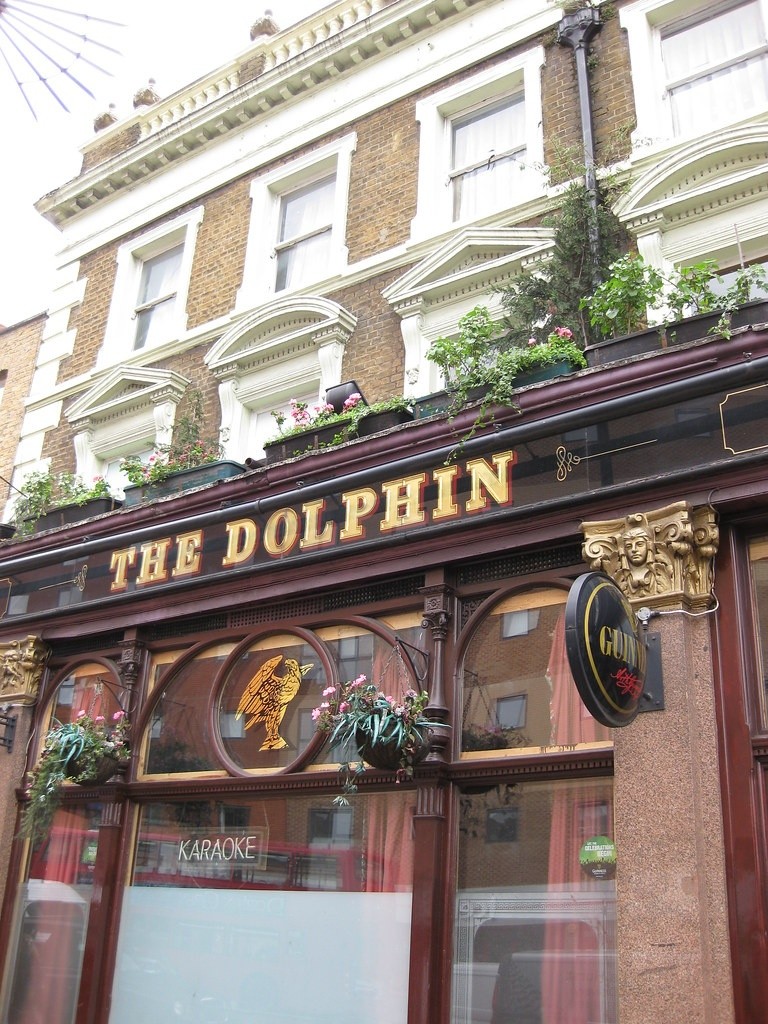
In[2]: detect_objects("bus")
[26,824,396,929]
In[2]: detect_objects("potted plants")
[576,247,768,369]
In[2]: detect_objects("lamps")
[324,381,369,416]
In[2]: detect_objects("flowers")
[426,306,587,468]
[262,395,424,459]
[118,439,221,489]
[459,720,532,839]
[12,708,134,845]
[6,463,110,542]
[313,673,436,806]
[147,743,223,856]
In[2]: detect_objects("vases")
[354,722,435,772]
[446,355,582,404]
[460,743,498,795]
[0,523,17,543]
[123,459,247,508]
[58,751,115,787]
[22,497,123,536]
[263,407,416,463]
